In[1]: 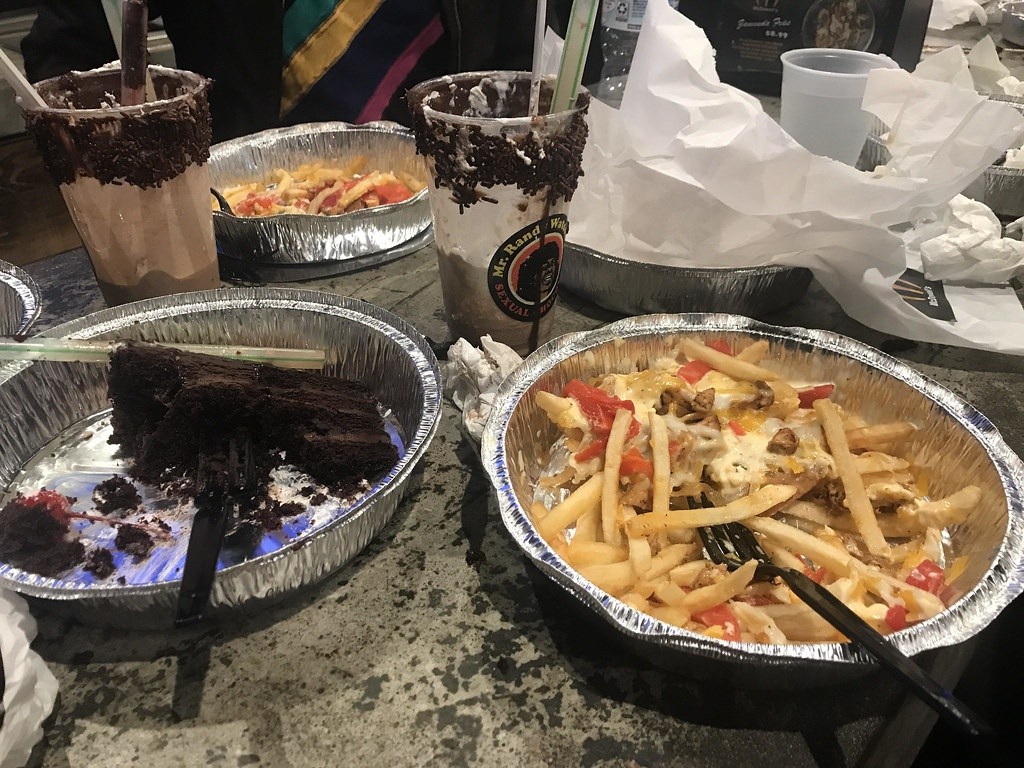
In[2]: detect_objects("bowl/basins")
[997,2,1024,48]
[0,287,444,630]
[0,257,41,345]
[559,240,813,316]
[861,91,1024,216]
[202,121,434,264]
[480,312,1024,668]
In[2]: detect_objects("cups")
[15,59,221,308]
[405,68,592,363]
[779,48,902,169]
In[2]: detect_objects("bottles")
[597,0,679,110]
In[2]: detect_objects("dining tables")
[0,94,1024,768]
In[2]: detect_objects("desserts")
[109,342,402,488]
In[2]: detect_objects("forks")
[685,491,989,738]
[173,439,254,629]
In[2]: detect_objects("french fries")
[211,168,421,218]
[533,338,982,642]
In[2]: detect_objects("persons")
[19,0,605,146]
